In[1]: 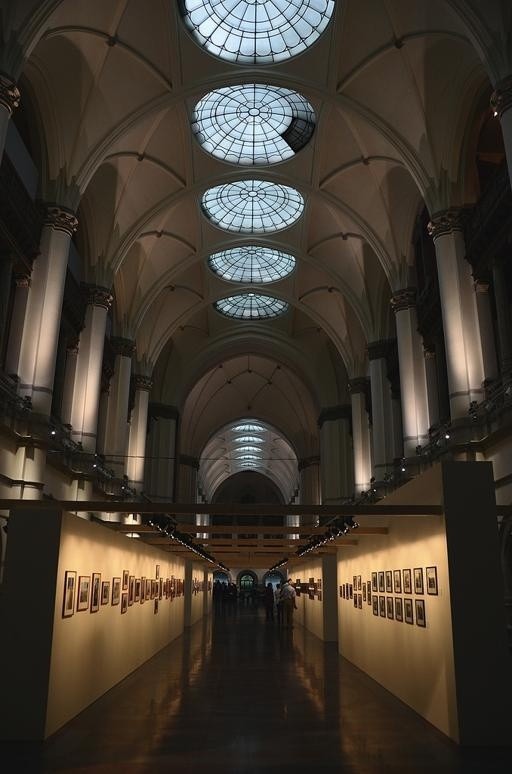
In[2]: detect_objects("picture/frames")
[61,565,163,618]
[352,566,439,628]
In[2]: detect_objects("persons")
[213,578,298,629]
